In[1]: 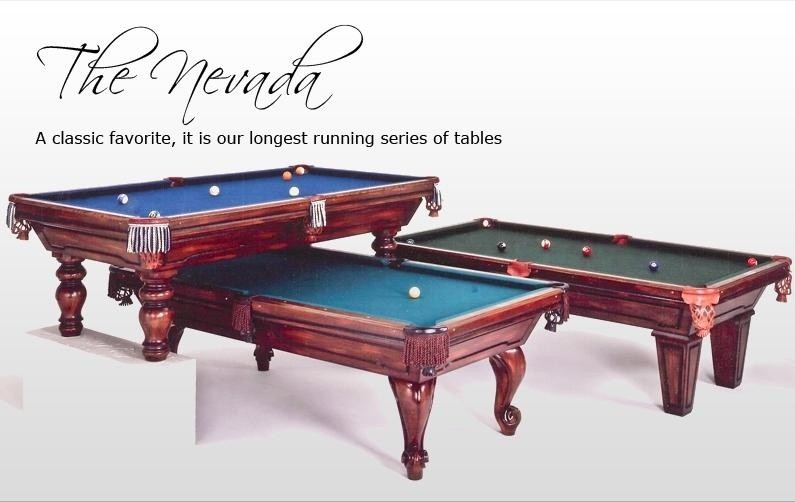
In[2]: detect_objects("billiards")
[116,193,128,204]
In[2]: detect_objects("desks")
[6,165,792,480]
